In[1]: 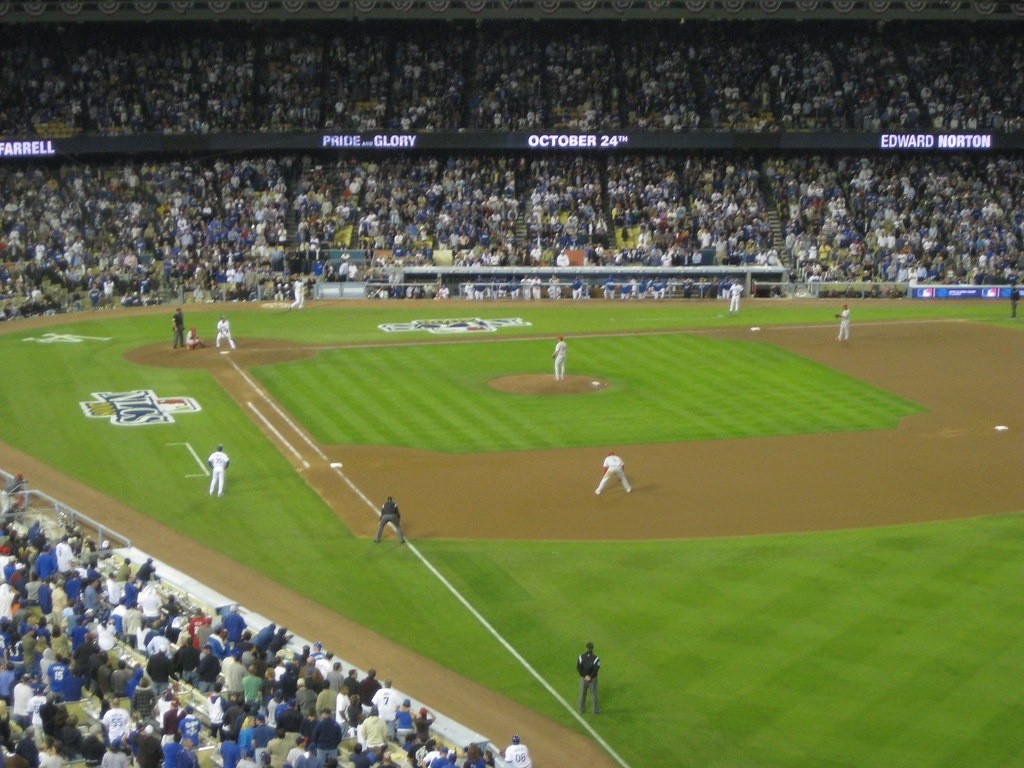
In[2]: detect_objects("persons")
[728,279,744,312]
[0,473,532,768]
[216,315,235,350]
[835,304,850,342]
[290,278,305,310]
[552,336,566,381]
[0,27,1024,318]
[186,326,210,350]
[374,496,405,544]
[1010,283,1020,318]
[208,445,229,497]
[577,643,601,715]
[593,451,632,495]
[172,308,185,349]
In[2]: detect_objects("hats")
[177,307,181,311]
[320,708,331,713]
[449,754,456,762]
[440,748,448,755]
[288,699,296,705]
[295,736,307,745]
[607,451,614,456]
[843,304,848,307]
[147,558,152,563]
[558,335,563,341]
[0,517,305,767]
[314,642,322,651]
[419,707,427,713]
[403,699,410,706]
[190,326,196,331]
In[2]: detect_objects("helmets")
[512,735,520,745]
[217,445,222,451]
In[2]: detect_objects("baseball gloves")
[552,354,555,358]
[835,314,839,318]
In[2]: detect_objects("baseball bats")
[226,333,237,345]
